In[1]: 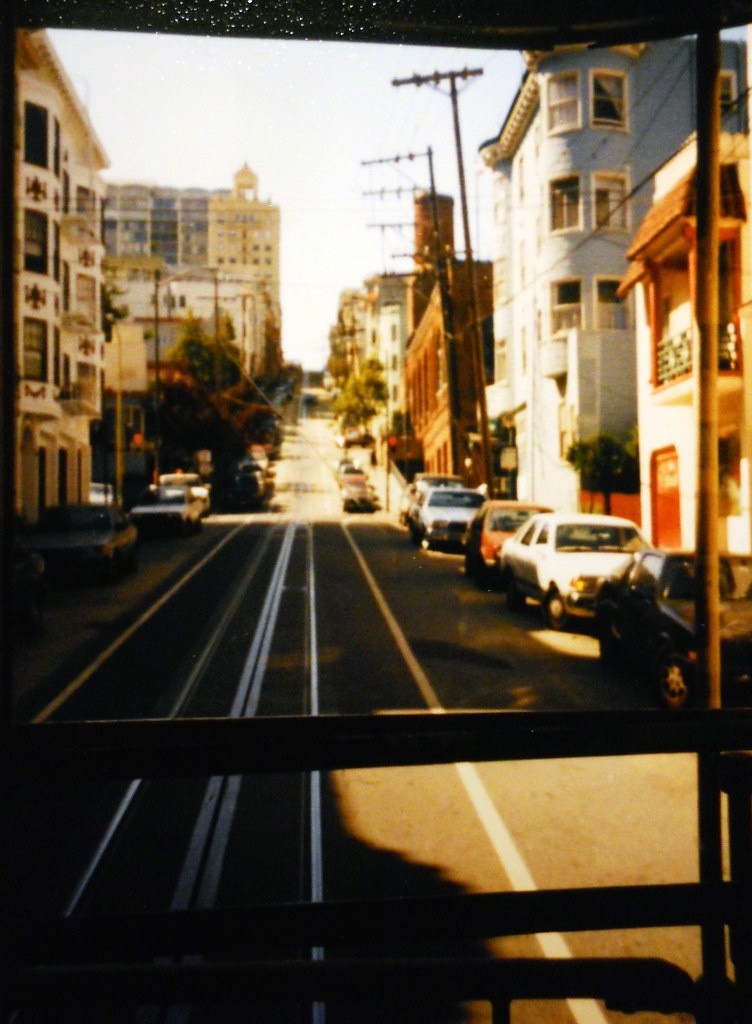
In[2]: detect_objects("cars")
[337,458,373,511]
[232,421,276,498]
[500,512,655,630]
[595,549,752,708]
[17,503,140,576]
[129,483,204,531]
[459,500,556,573]
[149,466,212,515]
[399,472,490,548]
[337,431,374,448]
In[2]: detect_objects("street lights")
[201,265,223,483]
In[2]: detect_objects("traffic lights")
[129,434,143,454]
